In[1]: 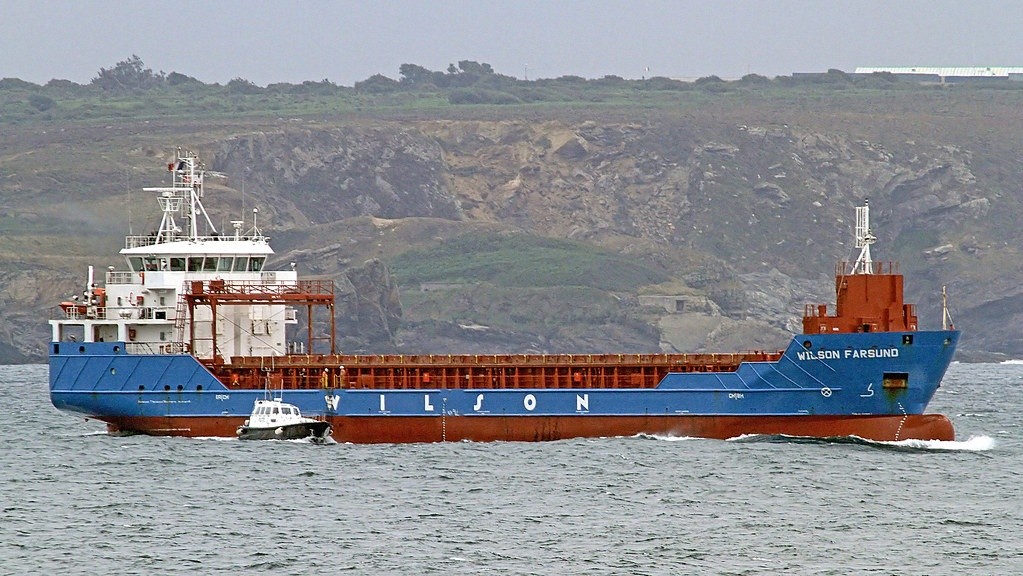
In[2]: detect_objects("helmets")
[339,366,344,369]
[325,368,329,373]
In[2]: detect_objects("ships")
[47,145,961,443]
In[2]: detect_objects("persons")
[340,365,346,390]
[322,368,328,386]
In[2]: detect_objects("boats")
[236,378,331,441]
